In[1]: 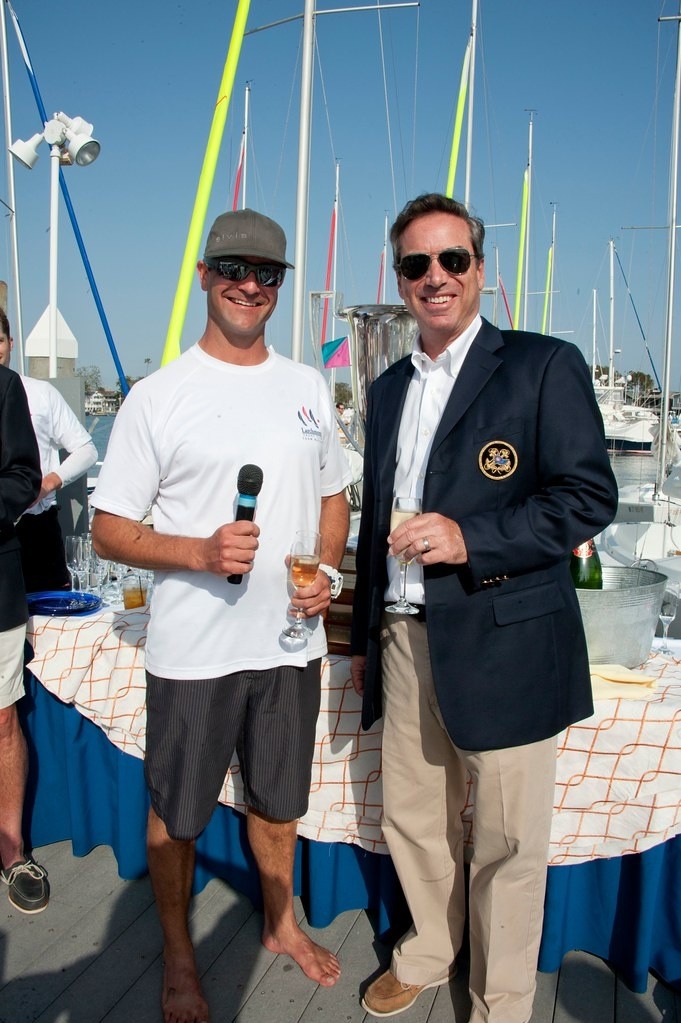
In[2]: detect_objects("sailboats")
[0,0,681,610]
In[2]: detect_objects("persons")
[261,268,279,286]
[336,402,351,443]
[349,194,618,1023]
[229,264,241,281]
[89,209,353,1023]
[0,365,50,914]
[0,309,99,591]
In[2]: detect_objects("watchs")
[319,564,344,599]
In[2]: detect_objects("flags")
[321,337,351,368]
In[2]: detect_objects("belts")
[386,602,426,622]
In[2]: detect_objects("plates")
[24,587,104,616]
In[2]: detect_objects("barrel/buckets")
[572,565,668,670]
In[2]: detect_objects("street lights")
[8,112,102,384]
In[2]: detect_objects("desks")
[18,574,681,998]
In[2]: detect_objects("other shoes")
[0,853,50,914]
[361,953,458,1017]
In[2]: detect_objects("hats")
[204,208,295,269]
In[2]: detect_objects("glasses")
[205,256,285,286]
[398,248,478,280]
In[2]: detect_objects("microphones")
[227,464,263,584]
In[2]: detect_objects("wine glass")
[281,528,322,637]
[66,528,157,608]
[659,579,679,655]
[384,495,422,615]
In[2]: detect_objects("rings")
[423,538,430,550]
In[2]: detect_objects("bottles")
[569,532,602,590]
